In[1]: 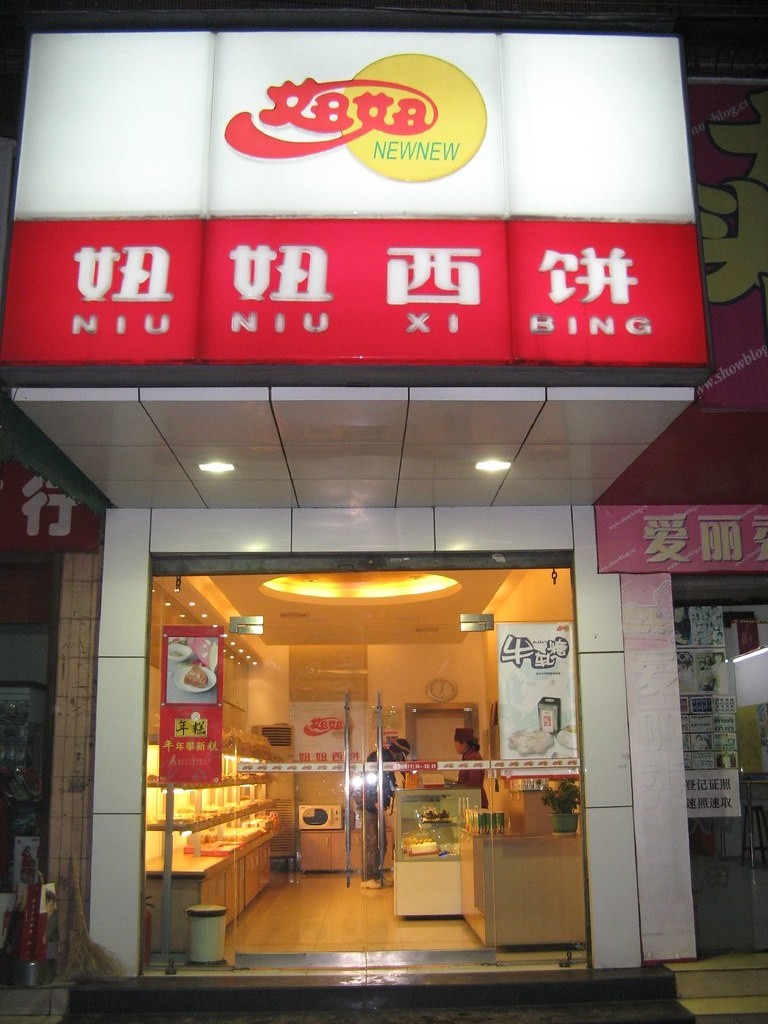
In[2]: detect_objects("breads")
[147,733,275,845]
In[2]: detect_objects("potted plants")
[541,777,580,836]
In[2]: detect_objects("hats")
[454,728,474,741]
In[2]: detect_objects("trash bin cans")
[185,902,227,964]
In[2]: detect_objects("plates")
[168,643,192,662]
[173,666,216,693]
[556,729,577,749]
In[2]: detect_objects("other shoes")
[379,876,395,886]
[362,878,385,889]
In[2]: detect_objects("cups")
[511,778,549,790]
[565,728,577,742]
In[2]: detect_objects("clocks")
[427,678,457,702]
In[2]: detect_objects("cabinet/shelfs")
[300,828,393,877]
[144,743,280,953]
[459,826,588,945]
[394,787,482,916]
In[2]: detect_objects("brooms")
[52,849,124,984]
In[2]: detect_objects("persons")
[674,607,737,769]
[43,891,59,959]
[350,739,410,888]
[454,728,489,809]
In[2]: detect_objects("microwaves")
[299,805,342,829]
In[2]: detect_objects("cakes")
[411,838,438,854]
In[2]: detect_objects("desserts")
[421,806,450,822]
[183,665,208,688]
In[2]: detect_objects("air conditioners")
[250,725,297,873]
